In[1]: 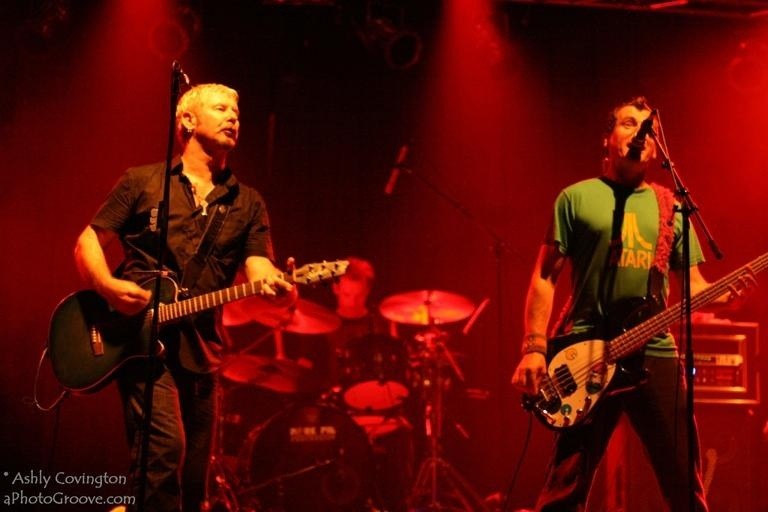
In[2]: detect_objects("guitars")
[50,261,348,394]
[519,251,766,430]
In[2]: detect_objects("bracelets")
[521,334,549,357]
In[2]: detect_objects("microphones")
[625,108,664,160]
[383,145,408,195]
[174,60,188,88]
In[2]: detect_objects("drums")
[240,404,374,510]
[336,333,407,411]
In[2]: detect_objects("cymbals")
[227,352,319,393]
[381,290,474,325]
[256,297,339,334]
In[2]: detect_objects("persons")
[72,81,300,507]
[509,95,757,511]
[325,254,389,352]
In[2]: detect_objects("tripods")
[411,376,476,511]
